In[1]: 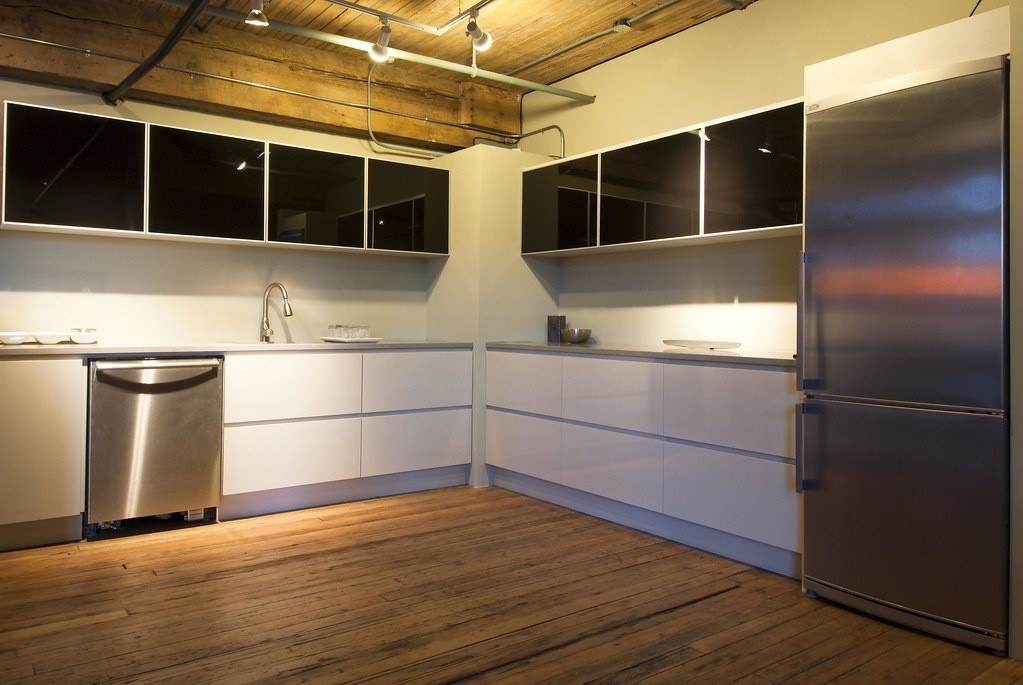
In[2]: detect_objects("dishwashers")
[88,354,223,542]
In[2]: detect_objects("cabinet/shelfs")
[0,100,450,257]
[485,348,805,580]
[220,349,473,522]
[0,354,88,554]
[520,95,804,258]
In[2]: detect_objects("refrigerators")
[804,55,1015,657]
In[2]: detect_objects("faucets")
[260,282,293,344]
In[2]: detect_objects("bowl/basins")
[560,329,592,344]
[0,332,99,344]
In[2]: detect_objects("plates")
[319,337,381,343]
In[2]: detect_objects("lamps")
[244,0,271,26]
[369,16,392,62]
[465,10,492,52]
